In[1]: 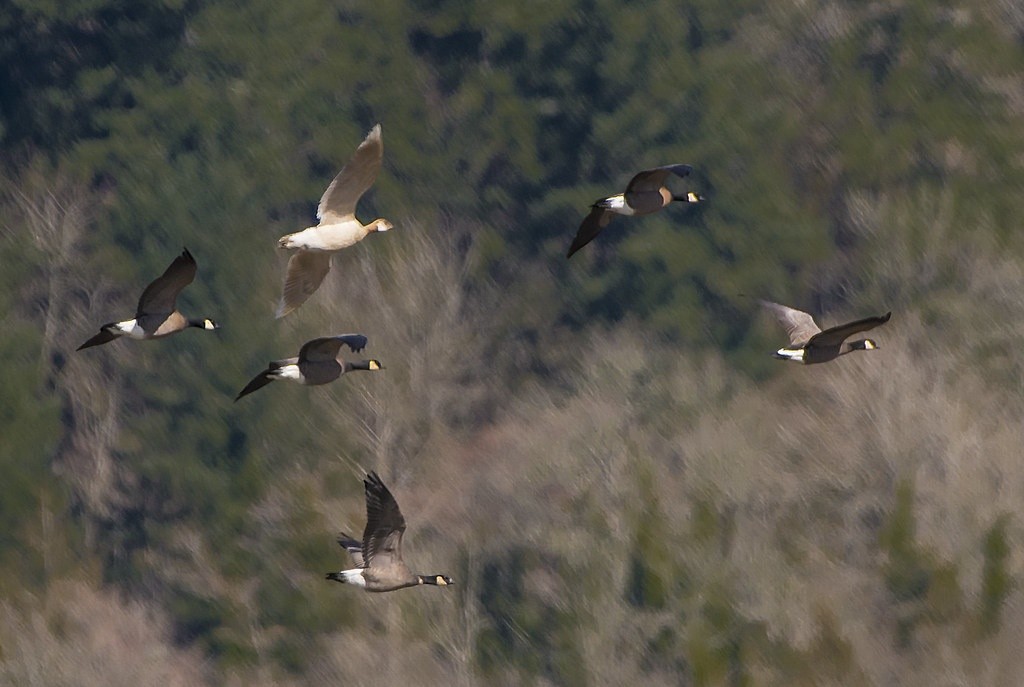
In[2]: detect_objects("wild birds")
[323,468,457,594]
[565,162,707,263]
[272,120,396,321]
[734,290,893,367]
[74,244,224,354]
[231,332,391,407]
